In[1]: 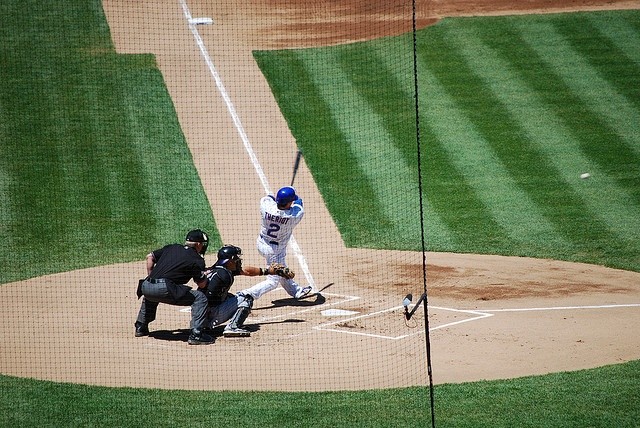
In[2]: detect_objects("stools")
[148,278,166,284]
[260,235,278,245]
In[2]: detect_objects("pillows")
[186,229,209,257]
[216,244,243,272]
[276,186,298,205]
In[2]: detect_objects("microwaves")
[291,151,302,186]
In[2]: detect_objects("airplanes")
[268,262,295,279]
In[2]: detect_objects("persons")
[134,229,215,345]
[197,244,295,336]
[237,186,312,300]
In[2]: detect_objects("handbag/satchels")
[259,268,268,276]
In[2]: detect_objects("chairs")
[188,327,215,345]
[294,286,312,300]
[236,292,244,295]
[135,321,149,337]
[223,327,251,337]
